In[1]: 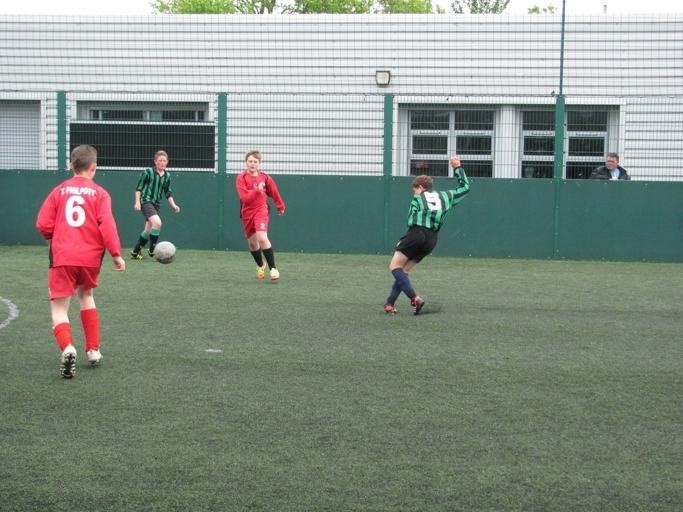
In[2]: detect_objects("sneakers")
[60,345,77,380]
[256,261,265,278]
[85,349,104,369]
[269,268,279,279]
[129,249,154,259]
[384,296,425,315]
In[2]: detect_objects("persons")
[130,150,181,260]
[589,153,630,181]
[36,145,125,378]
[383,156,470,314]
[235,150,285,280]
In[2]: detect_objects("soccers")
[154,241,176,263]
